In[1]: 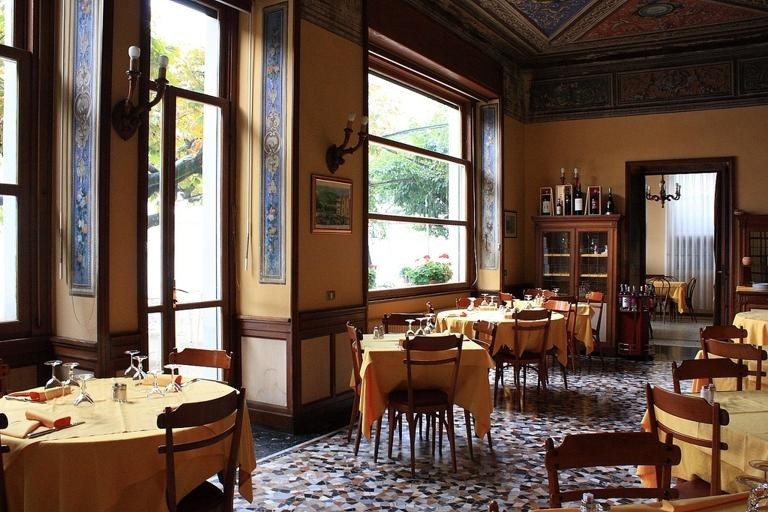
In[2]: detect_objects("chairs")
[157,387,246,512]
[168,348,235,384]
[383,311,437,459]
[386,333,466,481]
[542,428,682,509]
[345,320,384,465]
[647,274,698,323]
[460,320,500,461]
[648,309,767,500]
[427,288,604,396]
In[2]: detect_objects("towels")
[1,408,71,440]
[8,387,73,400]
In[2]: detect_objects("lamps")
[113,44,170,140]
[326,110,369,174]
[645,174,681,208]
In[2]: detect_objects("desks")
[735,285,768,311]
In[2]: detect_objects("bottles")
[112,384,126,402]
[605,187,614,214]
[574,183,585,215]
[373,326,378,339]
[541,190,550,215]
[556,198,561,215]
[700,384,715,404]
[356,326,363,340]
[619,284,657,311]
[565,187,570,215]
[591,189,599,214]
[586,234,611,257]
[379,322,384,339]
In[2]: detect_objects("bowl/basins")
[753,282,767,289]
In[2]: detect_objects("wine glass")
[467,288,560,315]
[405,312,436,338]
[43,360,97,419]
[124,350,185,412]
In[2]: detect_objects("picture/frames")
[503,210,518,239]
[309,173,354,236]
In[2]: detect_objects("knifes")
[180,377,199,387]
[28,421,84,438]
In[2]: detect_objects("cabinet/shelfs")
[619,298,654,360]
[529,215,629,354]
